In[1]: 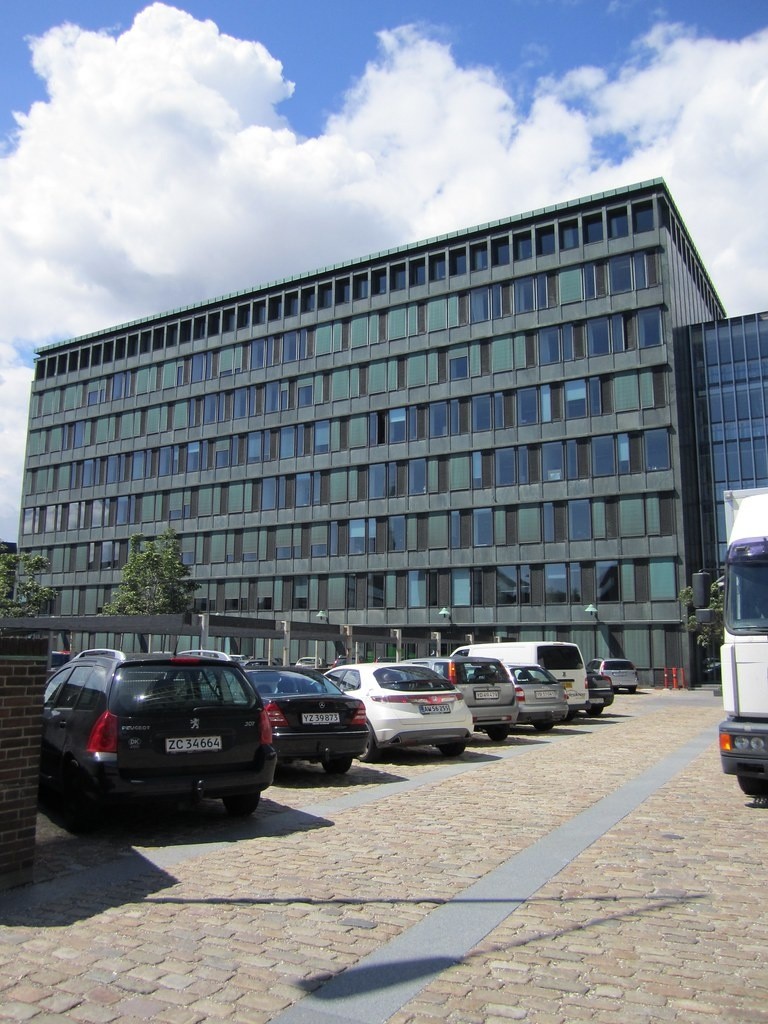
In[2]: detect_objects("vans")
[438,642,591,722]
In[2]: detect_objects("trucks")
[690,486,768,797]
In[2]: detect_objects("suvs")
[587,657,640,695]
[383,656,520,742]
[39,648,277,834]
[332,654,370,668]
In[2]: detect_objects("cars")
[466,662,570,734]
[199,663,370,777]
[375,654,404,663]
[701,656,722,684]
[585,668,616,718]
[296,656,327,669]
[229,655,282,668]
[308,663,476,764]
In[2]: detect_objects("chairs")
[473,668,485,678]
[383,673,402,683]
[518,671,528,679]
[257,684,272,693]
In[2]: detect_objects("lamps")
[316,610,329,620]
[437,608,450,618]
[585,604,598,616]
